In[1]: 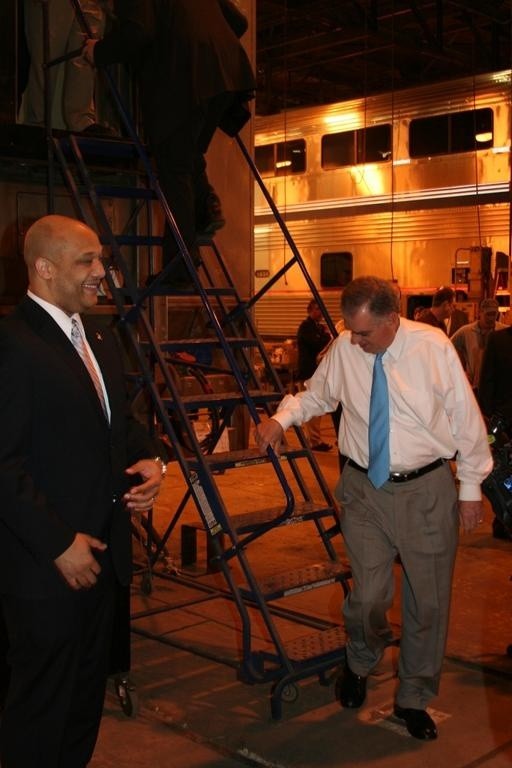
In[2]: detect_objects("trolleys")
[37,1,398,727]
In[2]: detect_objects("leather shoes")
[393,706,438,741]
[334,666,365,709]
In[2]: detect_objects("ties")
[71,319,108,425]
[368,352,390,489]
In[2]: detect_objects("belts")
[349,458,446,483]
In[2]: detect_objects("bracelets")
[154,456,167,477]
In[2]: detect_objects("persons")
[295,300,334,454]
[1,214,167,768]
[79,0,255,288]
[408,283,512,541]
[252,269,495,742]
[20,1,113,136]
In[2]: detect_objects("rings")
[151,496,158,503]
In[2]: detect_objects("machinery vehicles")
[449,243,512,327]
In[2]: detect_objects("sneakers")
[491,517,511,540]
[312,442,333,451]
